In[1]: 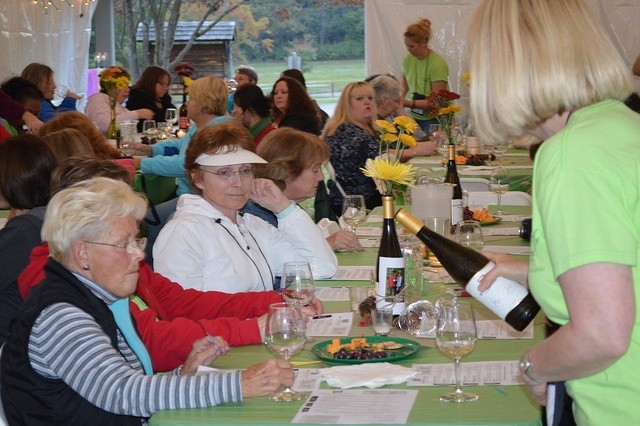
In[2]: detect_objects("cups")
[118,120,141,156]
[370,308,393,335]
[418,215,450,240]
[165,108,177,139]
[410,183,456,224]
[399,299,438,336]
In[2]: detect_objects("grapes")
[461,207,479,225]
[332,347,388,360]
[149,137,157,145]
[467,154,485,164]
[140,136,151,144]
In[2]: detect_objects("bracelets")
[412,99,416,108]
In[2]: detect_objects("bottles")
[393,207,544,333]
[107,105,121,151]
[179,92,191,133]
[375,195,406,324]
[444,143,464,235]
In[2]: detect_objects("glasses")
[234,76,252,81]
[156,79,170,89]
[195,165,254,179]
[81,238,147,254]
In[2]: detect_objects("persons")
[21,62,81,123]
[398,17,454,140]
[237,128,362,293]
[83,67,156,138]
[368,75,439,163]
[40,112,135,187]
[469,0,639,426]
[16,154,321,374]
[153,120,339,294]
[39,127,96,163]
[231,83,275,145]
[131,76,235,268]
[226,67,258,115]
[1,77,44,147]
[1,88,47,135]
[298,163,344,223]
[0,134,58,309]
[1,176,295,426]
[271,76,321,136]
[125,65,178,132]
[280,69,330,128]
[318,81,394,210]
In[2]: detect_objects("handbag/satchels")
[132,172,176,205]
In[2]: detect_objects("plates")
[480,216,502,226]
[310,335,421,363]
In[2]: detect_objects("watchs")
[520,351,544,384]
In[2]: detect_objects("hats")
[193,144,269,166]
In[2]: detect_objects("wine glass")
[454,221,484,254]
[280,261,315,305]
[122,136,137,156]
[54,85,84,106]
[265,303,308,402]
[435,301,479,403]
[342,195,366,252]
[428,124,442,155]
[143,120,157,144]
[226,79,238,91]
[489,169,510,215]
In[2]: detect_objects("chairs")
[446,176,492,190]
[467,191,531,205]
